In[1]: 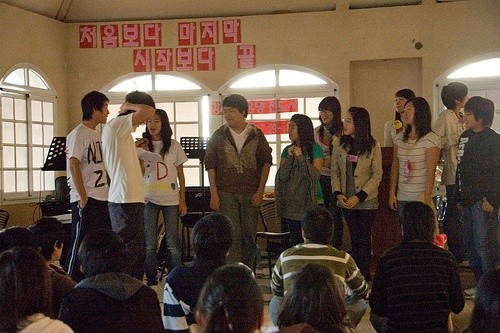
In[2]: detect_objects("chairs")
[0,209,9,229]
[259,200,277,230]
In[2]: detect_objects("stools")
[182,214,209,257]
[253,231,290,275]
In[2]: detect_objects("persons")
[276,114,325,245]
[389,97,442,245]
[65,90,110,279]
[142,109,187,291]
[102,91,157,282]
[269,210,370,328]
[331,107,383,288]
[277,264,355,333]
[462,267,500,333]
[384,88,417,147]
[434,82,469,266]
[0,212,263,333]
[452,96,500,299]
[312,96,344,211]
[204,94,273,271]
[369,202,465,333]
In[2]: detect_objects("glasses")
[319,109,331,114]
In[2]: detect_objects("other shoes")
[464,287,477,295]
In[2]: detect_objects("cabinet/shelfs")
[371,149,405,266]
